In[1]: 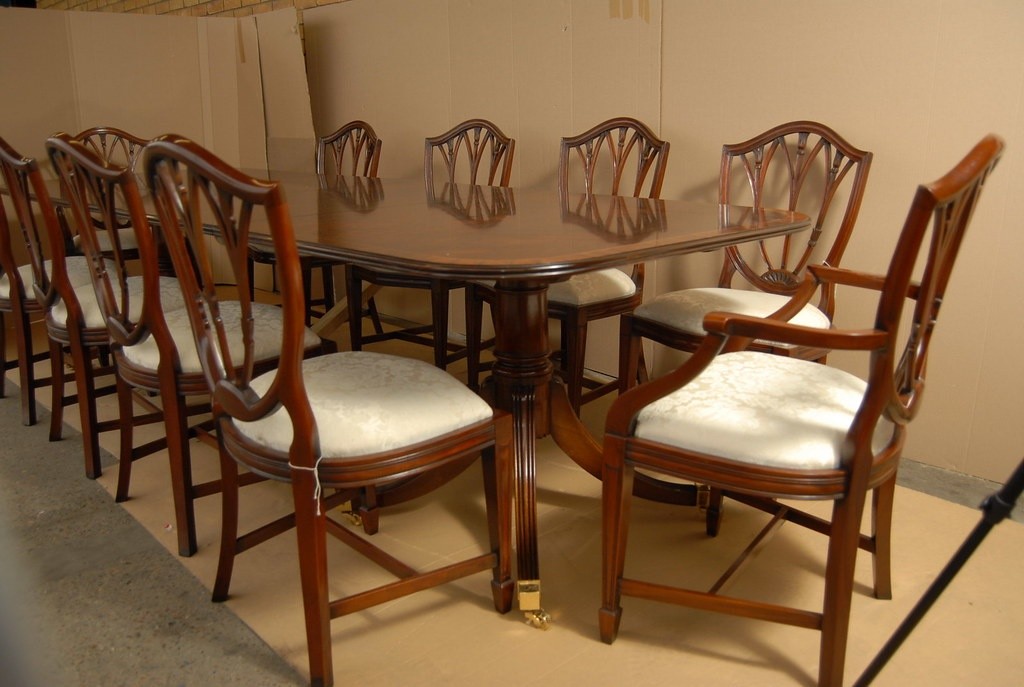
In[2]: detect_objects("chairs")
[0,118,1008,687]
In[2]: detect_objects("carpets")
[0,213,496,461]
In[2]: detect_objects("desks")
[0,165,810,631]
